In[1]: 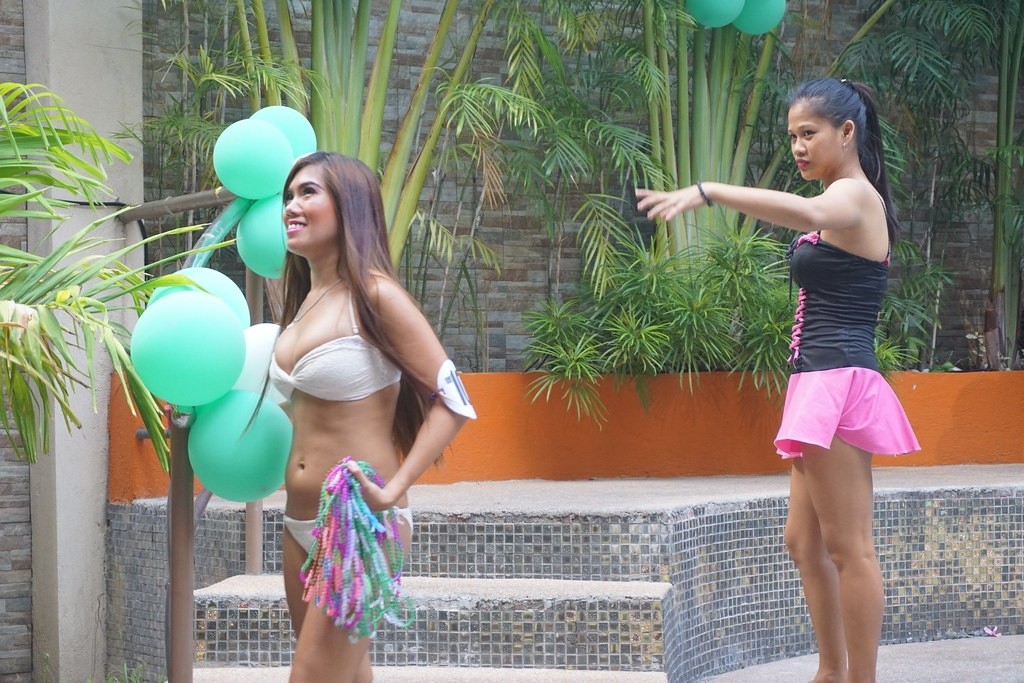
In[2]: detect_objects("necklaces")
[286,278,343,329]
[298,455,414,644]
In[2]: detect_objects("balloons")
[130,267,293,503]
[686,0,786,35]
[213,105,316,279]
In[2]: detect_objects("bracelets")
[696,181,713,207]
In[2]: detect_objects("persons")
[634,77,922,683]
[268,152,479,683]
